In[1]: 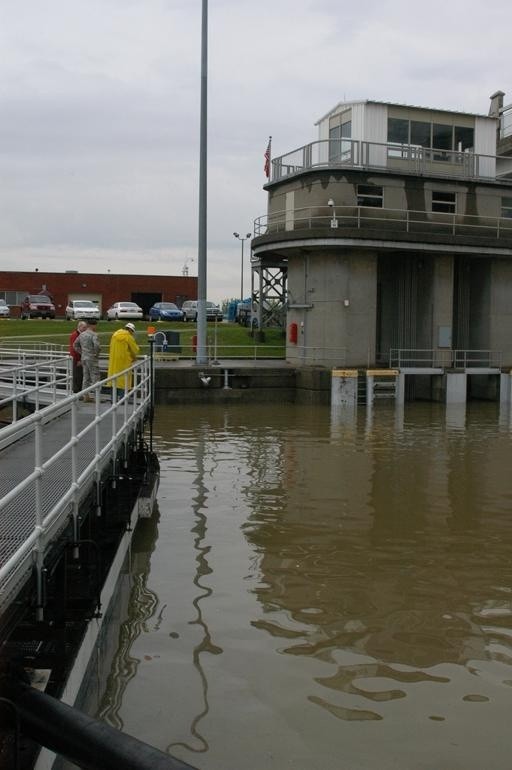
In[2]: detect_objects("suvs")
[21,295,56,320]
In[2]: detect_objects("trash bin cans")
[154,331,182,353]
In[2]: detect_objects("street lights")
[234,232,251,301]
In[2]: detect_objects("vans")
[182,301,222,322]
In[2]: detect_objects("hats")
[125,323,135,332]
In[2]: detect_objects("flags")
[264,140,271,177]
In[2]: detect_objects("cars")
[149,303,184,321]
[66,300,101,320]
[1,299,9,317]
[106,302,143,320]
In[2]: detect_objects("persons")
[73,320,106,403]
[107,323,140,405]
[70,320,87,400]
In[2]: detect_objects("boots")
[83,394,91,403]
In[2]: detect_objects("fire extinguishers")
[289,323,297,343]
